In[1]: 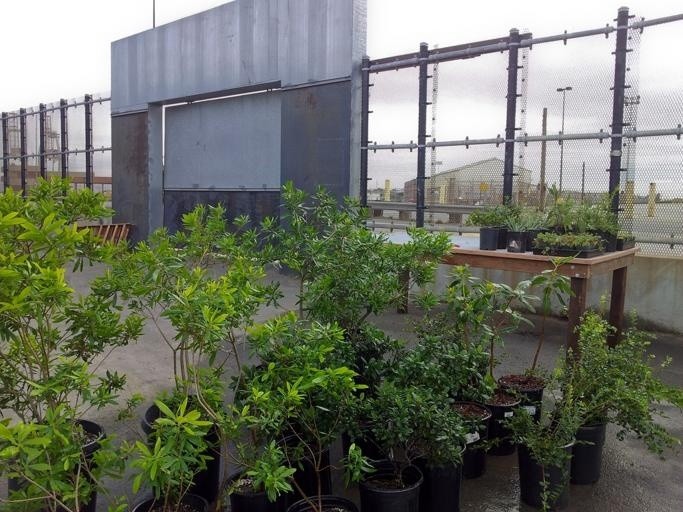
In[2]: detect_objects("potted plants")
[0,175,145,511]
[257,179,455,512]
[467,179,638,259]
[102,198,288,511]
[434,253,682,512]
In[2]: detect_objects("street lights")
[556,86,573,197]
[623,95,641,183]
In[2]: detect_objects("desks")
[390,242,643,370]
